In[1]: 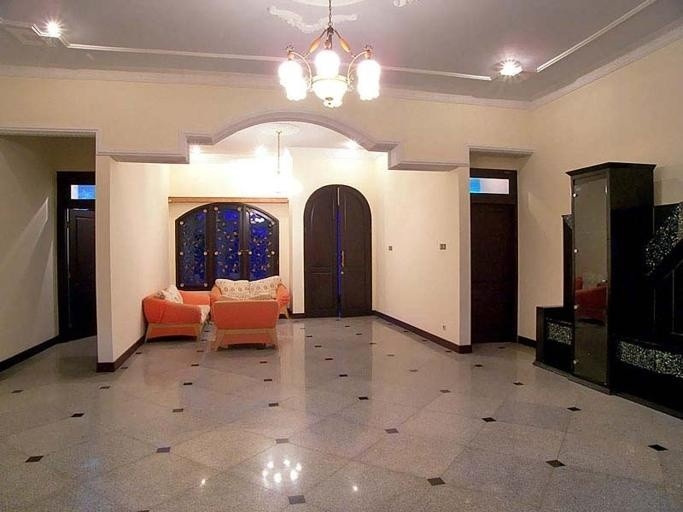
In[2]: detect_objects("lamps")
[276,1,383,110]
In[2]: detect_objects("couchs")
[210,275,290,320]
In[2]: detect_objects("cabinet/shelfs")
[560,213,571,321]
[566,162,660,393]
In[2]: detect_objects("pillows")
[250,274,282,296]
[213,277,250,300]
[156,284,185,304]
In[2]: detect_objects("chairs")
[208,296,281,349]
[141,285,209,342]
